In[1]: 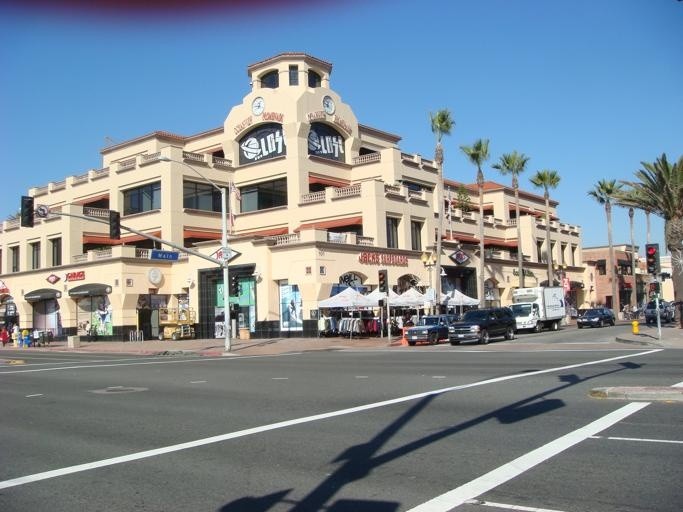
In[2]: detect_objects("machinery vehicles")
[156,303,196,341]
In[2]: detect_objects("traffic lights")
[645,244,661,274]
[649,282,660,293]
[109,209,120,240]
[377,269,388,292]
[228,272,239,298]
[19,195,34,228]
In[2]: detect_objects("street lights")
[157,156,230,352]
[419,250,436,287]
[553,258,567,285]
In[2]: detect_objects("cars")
[576,308,615,328]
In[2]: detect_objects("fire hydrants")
[631,320,640,335]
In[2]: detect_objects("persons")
[622,304,643,320]
[403,311,414,326]
[0,321,41,348]
[85,320,90,341]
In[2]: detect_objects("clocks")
[251,96,265,116]
[321,94,336,116]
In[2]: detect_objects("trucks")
[507,285,566,334]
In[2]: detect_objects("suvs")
[643,298,673,324]
[448,306,517,346]
[404,313,459,345]
